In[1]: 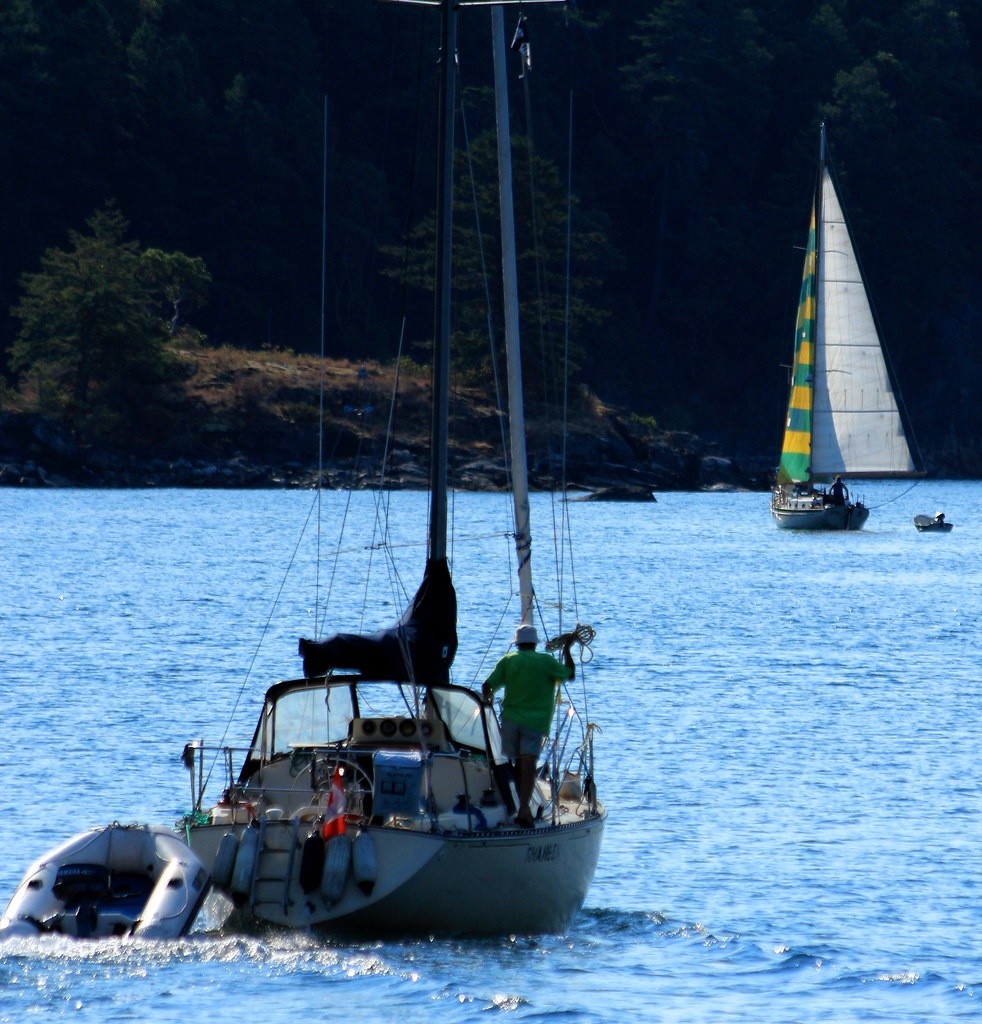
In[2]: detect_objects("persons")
[829,476,849,507]
[481,624,575,830]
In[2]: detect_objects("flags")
[322,765,347,841]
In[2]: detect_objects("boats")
[0,822,212,942]
[914,514,954,533]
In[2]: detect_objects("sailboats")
[173,1,608,937]
[772,124,929,531]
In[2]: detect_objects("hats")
[510,625,542,644]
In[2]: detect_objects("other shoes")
[513,806,535,829]
